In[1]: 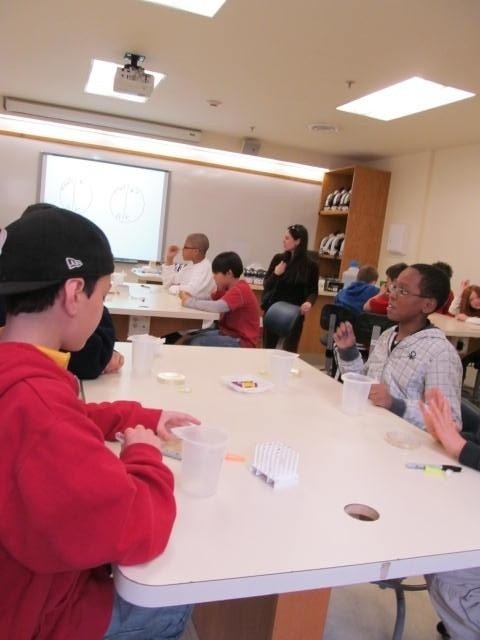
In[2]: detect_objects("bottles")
[343,261,361,292]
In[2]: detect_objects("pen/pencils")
[407,463,462,472]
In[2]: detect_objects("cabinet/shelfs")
[298,163,391,352]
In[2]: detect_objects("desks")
[427,313,480,349]
[71,343,480,640]
[100,282,220,339]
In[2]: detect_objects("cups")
[170,424,231,496]
[127,334,167,378]
[264,347,301,387]
[341,371,372,417]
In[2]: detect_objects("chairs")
[321,303,357,375]
[357,316,391,357]
[261,302,302,349]
[369,392,480,638]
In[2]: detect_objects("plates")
[223,374,278,395]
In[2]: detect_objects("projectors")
[114,67,155,98]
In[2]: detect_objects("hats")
[0,208,114,296]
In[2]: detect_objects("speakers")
[240,137,260,156]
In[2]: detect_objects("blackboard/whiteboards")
[37,151,171,264]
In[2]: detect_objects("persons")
[162,232,215,303]
[175,250,262,348]
[258,222,321,353]
[335,255,480,403]
[0,198,201,640]
[331,263,464,436]
[0,202,125,381]
[416,389,480,475]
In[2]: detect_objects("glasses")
[388,284,431,297]
